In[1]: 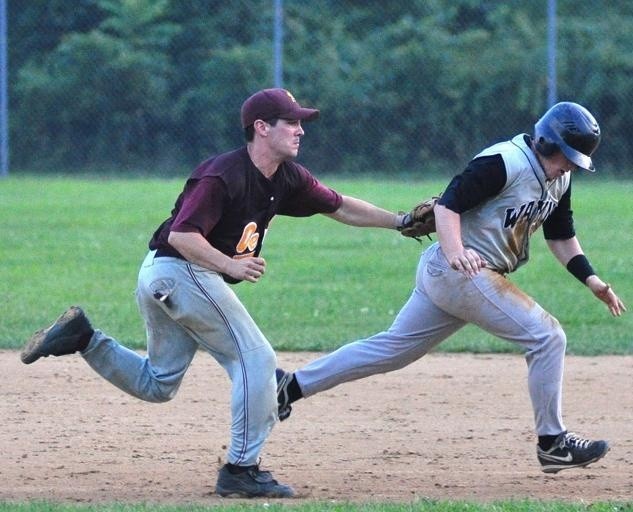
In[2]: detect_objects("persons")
[19,88,436,497]
[273,100,625,473]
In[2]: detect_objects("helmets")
[534,101,601,172]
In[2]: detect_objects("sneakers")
[536,431,608,473]
[20,306,93,364]
[216,464,294,498]
[274,368,293,422]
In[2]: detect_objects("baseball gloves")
[397,196,442,243]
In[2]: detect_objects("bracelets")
[566,255,593,283]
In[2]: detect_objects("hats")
[241,88,320,128]
